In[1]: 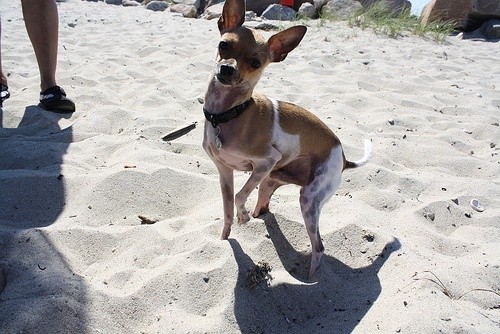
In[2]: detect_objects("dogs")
[203,0,374,280]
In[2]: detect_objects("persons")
[0,0,76,113]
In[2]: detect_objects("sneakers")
[40,86,75,113]
[0,83,10,106]
